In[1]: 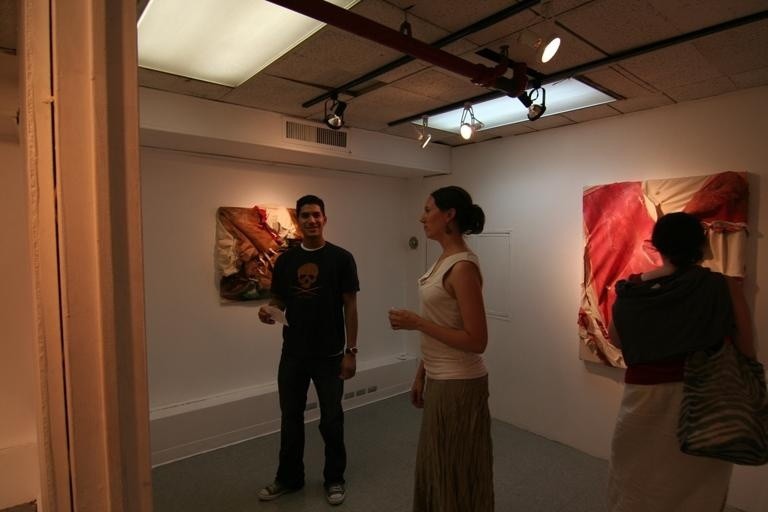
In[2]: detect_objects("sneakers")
[258,483,287,500]
[326,484,346,504]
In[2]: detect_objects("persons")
[389,187,497,511]
[257,194,361,506]
[606,211,757,512]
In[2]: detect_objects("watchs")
[346,347,358,356]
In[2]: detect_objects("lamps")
[460,121,480,140]
[518,29,562,64]
[325,101,347,130]
[518,86,545,121]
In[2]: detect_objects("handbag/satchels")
[673,272,768,466]
